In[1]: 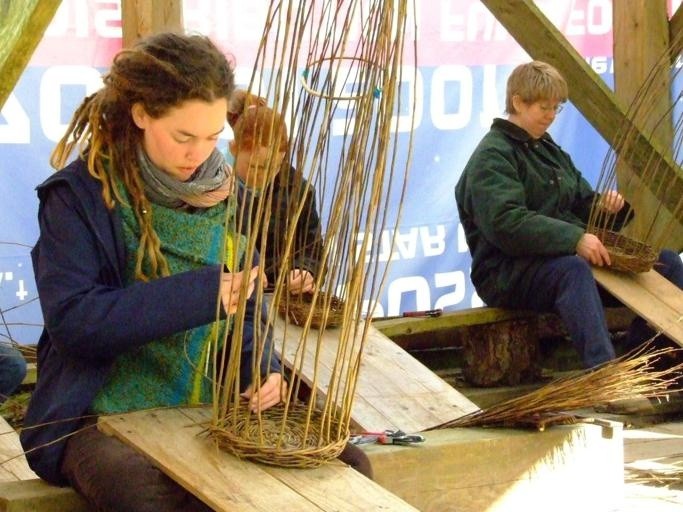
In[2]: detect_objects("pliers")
[350,428,425,445]
[403,309,442,317]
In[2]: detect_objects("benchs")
[2,481,84,511]
[370,303,552,385]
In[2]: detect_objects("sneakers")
[592,395,653,414]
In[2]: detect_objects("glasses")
[532,101,563,114]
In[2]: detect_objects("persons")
[0,345,27,407]
[19,33,372,512]
[455,60,683,415]
[226,90,328,296]
